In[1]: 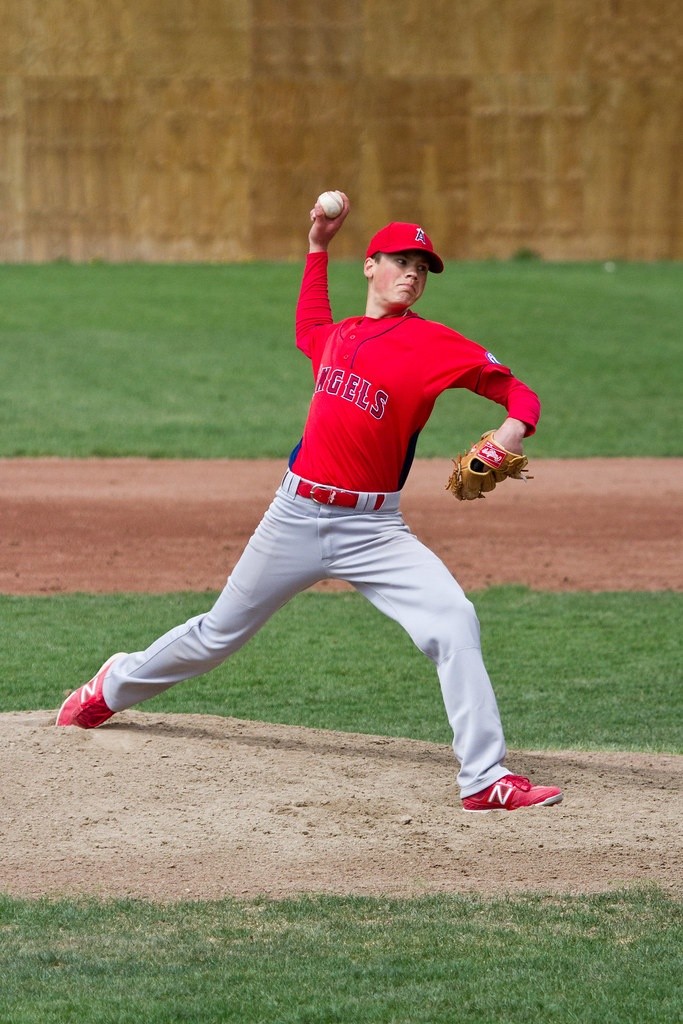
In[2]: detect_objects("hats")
[367,220,444,273]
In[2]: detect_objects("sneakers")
[461,772,564,814]
[55,651,127,730]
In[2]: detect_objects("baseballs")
[318,189,345,216]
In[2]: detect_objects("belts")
[296,482,385,510]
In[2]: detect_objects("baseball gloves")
[449,430,528,502]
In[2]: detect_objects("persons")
[55,190,566,814]
